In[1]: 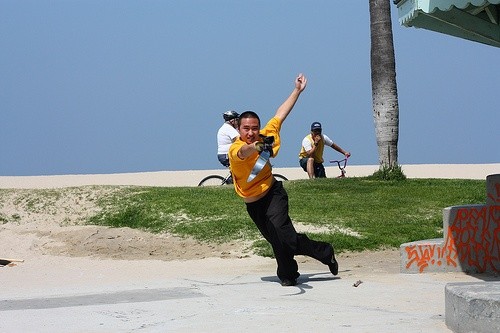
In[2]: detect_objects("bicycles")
[330,157,350,178]
[198,162,289,186]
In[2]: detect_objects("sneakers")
[328,255,338,275]
[281,278,297,285]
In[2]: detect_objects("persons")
[217,111,241,168]
[228,73,339,286]
[298,121,351,180]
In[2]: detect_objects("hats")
[311,121,321,132]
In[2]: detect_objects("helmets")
[223,110,239,121]
